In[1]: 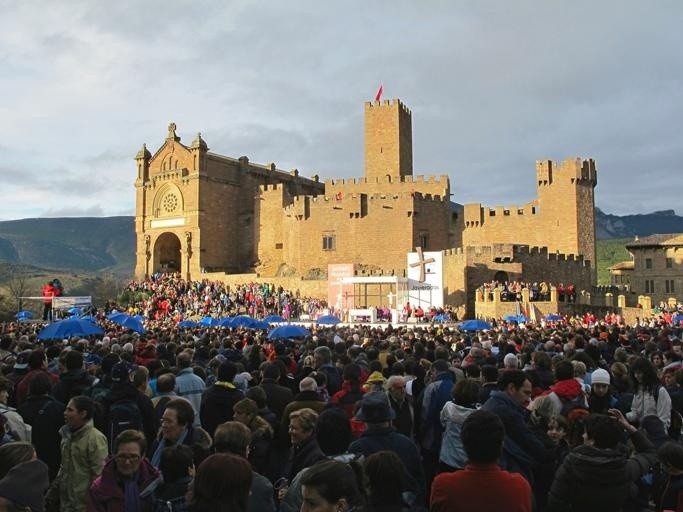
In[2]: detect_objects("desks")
[348,308,378,323]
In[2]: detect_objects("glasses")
[114,454,139,462]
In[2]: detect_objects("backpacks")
[104,392,145,453]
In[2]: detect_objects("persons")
[1,266,683,511]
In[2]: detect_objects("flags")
[376,85,383,100]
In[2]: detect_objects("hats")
[85,354,102,364]
[591,368,610,384]
[504,354,518,368]
[111,360,137,380]
[13,351,29,369]
[352,391,396,422]
